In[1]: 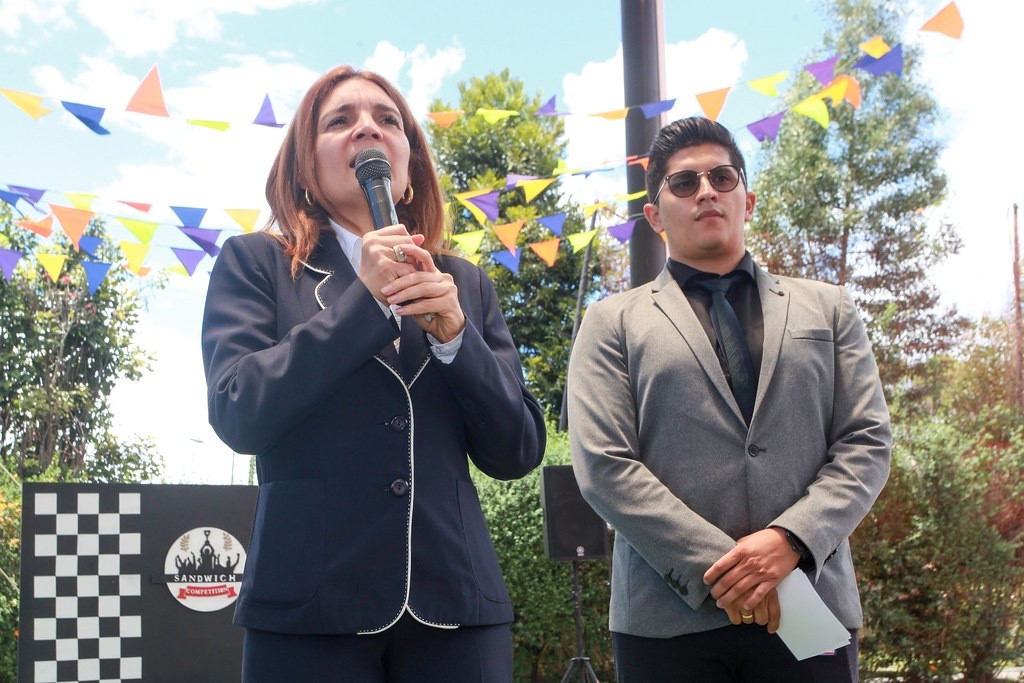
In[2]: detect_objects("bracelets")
[781,528,808,564]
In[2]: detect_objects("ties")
[685,271,758,427]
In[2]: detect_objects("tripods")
[561,563,600,683]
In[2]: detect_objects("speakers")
[541,464,609,563]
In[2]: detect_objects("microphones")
[356,149,415,305]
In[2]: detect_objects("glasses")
[652,164,747,204]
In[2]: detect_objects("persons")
[201,67,548,683]
[565,116,897,683]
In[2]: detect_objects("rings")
[393,243,408,264]
[742,614,753,619]
